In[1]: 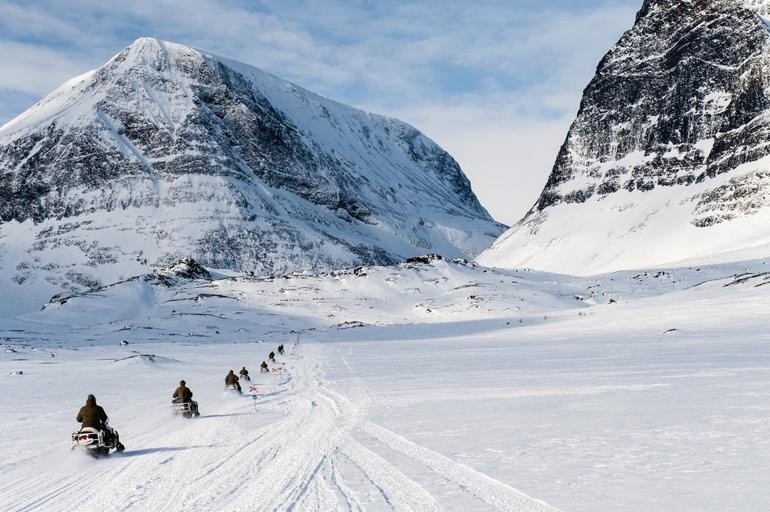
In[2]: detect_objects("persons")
[225,369,239,385]
[281,343,284,350]
[269,351,274,358]
[261,361,268,368]
[76,394,113,446]
[173,380,193,402]
[278,345,282,352]
[239,366,248,378]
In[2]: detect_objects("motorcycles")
[71,416,115,461]
[223,350,285,394]
[170,398,199,420]
